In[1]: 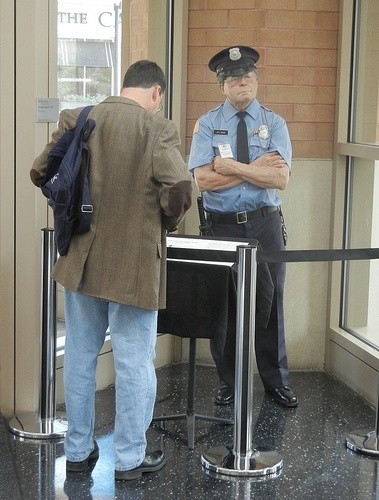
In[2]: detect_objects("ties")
[236,111,249,165]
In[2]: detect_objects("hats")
[208,45,260,77]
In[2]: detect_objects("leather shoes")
[115,450,166,479]
[66,440,100,471]
[214,385,234,405]
[265,385,299,407]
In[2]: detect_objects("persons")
[30,60,192,480]
[188,44,298,407]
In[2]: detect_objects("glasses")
[157,93,164,112]
[225,73,255,85]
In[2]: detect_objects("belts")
[206,206,279,224]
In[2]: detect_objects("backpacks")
[41,105,96,256]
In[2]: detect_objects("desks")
[152,234,275,450]
